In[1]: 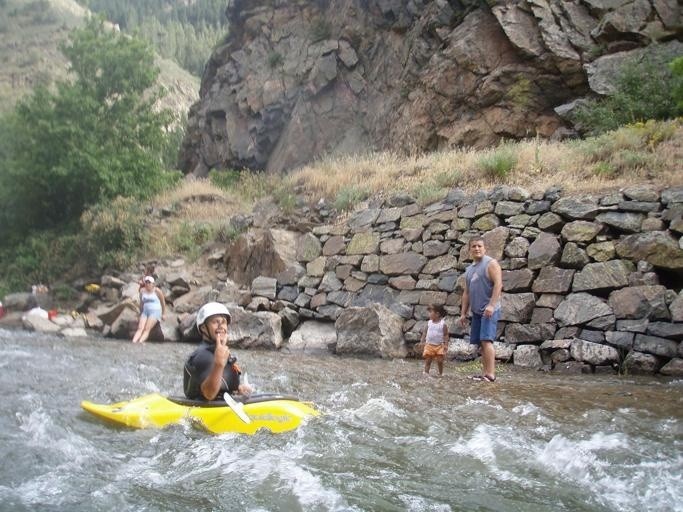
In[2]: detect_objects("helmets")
[196,302,232,336]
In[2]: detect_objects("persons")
[183,301,255,401]
[131,275,167,344]
[419,302,451,377]
[459,237,502,383]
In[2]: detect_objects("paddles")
[224,370,252,423]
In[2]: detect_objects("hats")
[144,276,154,283]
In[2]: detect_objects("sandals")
[468,374,497,382]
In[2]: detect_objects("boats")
[78,392,322,439]
